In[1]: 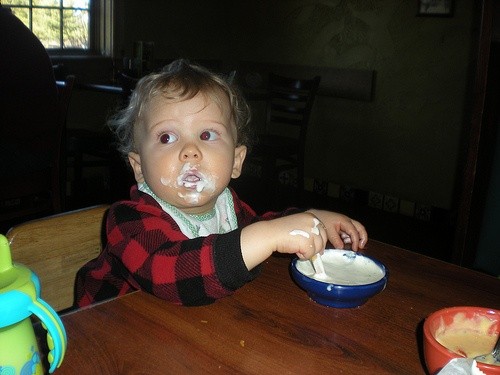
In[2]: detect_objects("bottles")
[0,234,67,375]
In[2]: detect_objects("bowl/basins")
[290,249,389,307]
[424,306,500,375]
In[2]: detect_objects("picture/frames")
[416,0,455,17]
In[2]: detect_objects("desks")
[50,236,500,375]
[75,80,130,109]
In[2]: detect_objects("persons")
[76,59,368,307]
[0,5,59,209]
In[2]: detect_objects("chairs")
[0,47,120,320]
[239,74,322,210]
